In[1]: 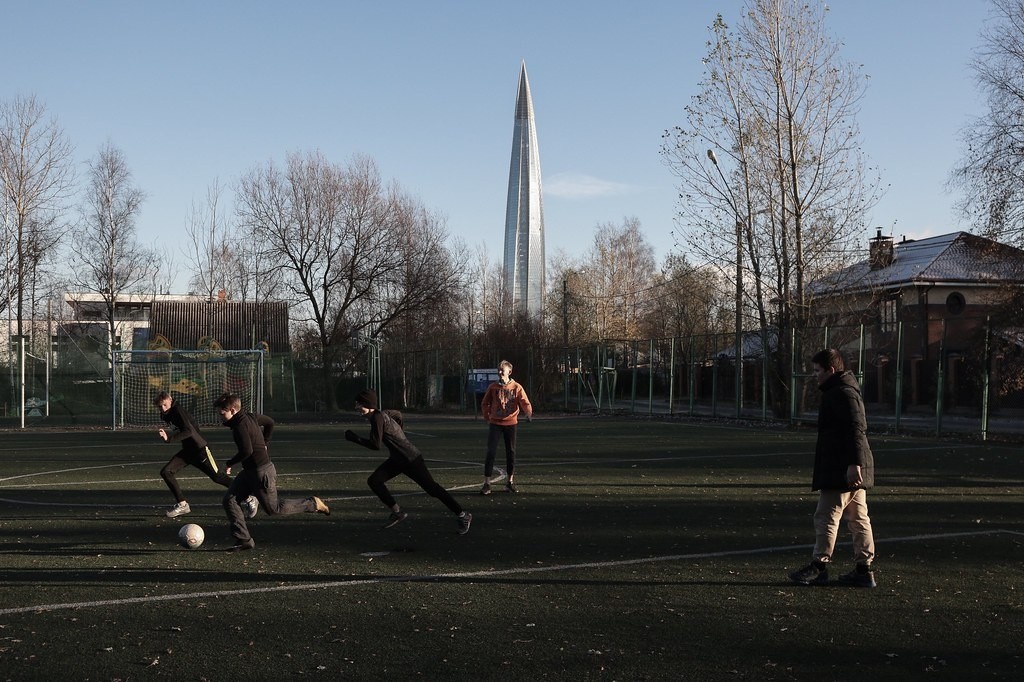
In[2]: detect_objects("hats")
[354,388,378,409]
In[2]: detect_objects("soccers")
[178,523,206,550]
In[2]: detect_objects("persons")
[792,350,877,588]
[481,360,532,495]
[344,389,472,535]
[213,392,330,551]
[153,391,259,518]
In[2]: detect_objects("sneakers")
[481,483,491,495]
[383,506,408,528]
[789,562,833,586]
[839,569,876,588]
[506,481,519,495]
[455,511,472,535]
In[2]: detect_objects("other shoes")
[309,496,331,516]
[245,495,259,518]
[227,538,255,553]
[166,502,191,517]
